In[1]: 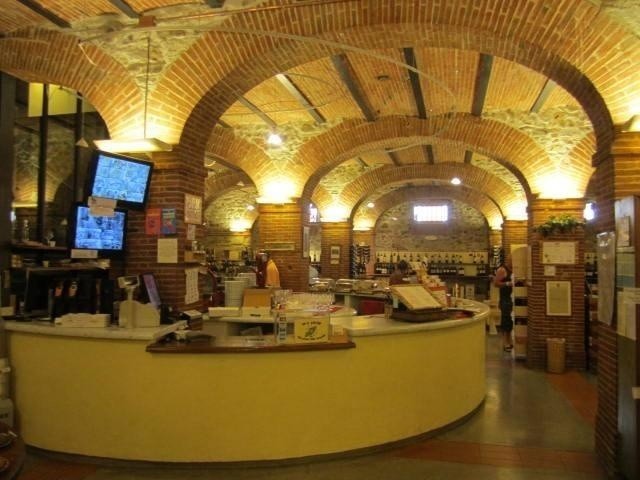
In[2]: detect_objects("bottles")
[349,245,503,278]
[205,248,257,279]
[309,254,321,277]
[20,219,30,244]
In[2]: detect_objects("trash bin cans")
[546,338,566,374]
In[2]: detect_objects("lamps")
[267,76,281,145]
[93,31,173,153]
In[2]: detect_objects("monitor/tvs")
[87,150,153,210]
[68,202,127,254]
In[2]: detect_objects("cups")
[275,290,335,311]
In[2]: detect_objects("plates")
[8,253,72,270]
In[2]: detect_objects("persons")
[309,255,319,287]
[493,254,515,352]
[388,259,409,285]
[249,247,281,288]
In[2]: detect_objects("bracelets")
[506,282,510,287]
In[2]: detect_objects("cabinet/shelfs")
[511,274,528,362]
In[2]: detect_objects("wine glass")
[44,230,54,246]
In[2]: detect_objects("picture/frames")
[539,240,579,266]
[545,280,572,317]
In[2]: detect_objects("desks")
[11,243,69,314]
[457,276,490,300]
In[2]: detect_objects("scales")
[118,275,142,330]
